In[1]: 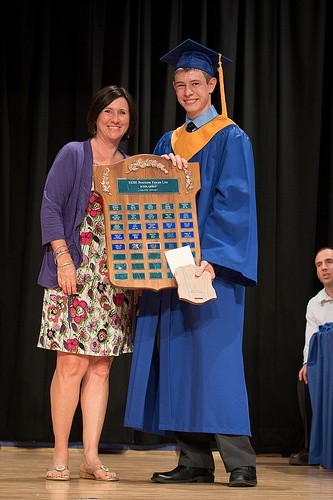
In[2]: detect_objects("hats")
[160,39,234,118]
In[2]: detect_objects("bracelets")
[52,245,70,265]
[57,261,75,269]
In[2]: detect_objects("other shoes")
[289,450,309,465]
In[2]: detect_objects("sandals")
[46,466,71,480]
[78,461,118,481]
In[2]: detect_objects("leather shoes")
[152,463,215,484]
[229,465,256,485]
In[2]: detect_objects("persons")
[124,40,258,488]
[288,249,333,467]
[36,85,133,482]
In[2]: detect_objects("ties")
[187,122,196,132]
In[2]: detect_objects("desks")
[307,322,333,471]
